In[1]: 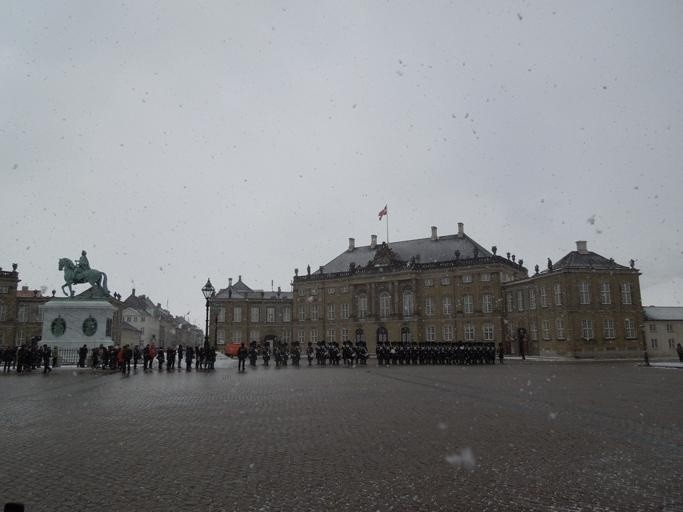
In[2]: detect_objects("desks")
[494,296,507,344]
[199,277,215,371]
[638,324,651,366]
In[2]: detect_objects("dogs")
[58,257,110,297]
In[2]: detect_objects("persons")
[676,342,682,362]
[72,250,88,285]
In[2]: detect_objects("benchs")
[377,205,386,220]
